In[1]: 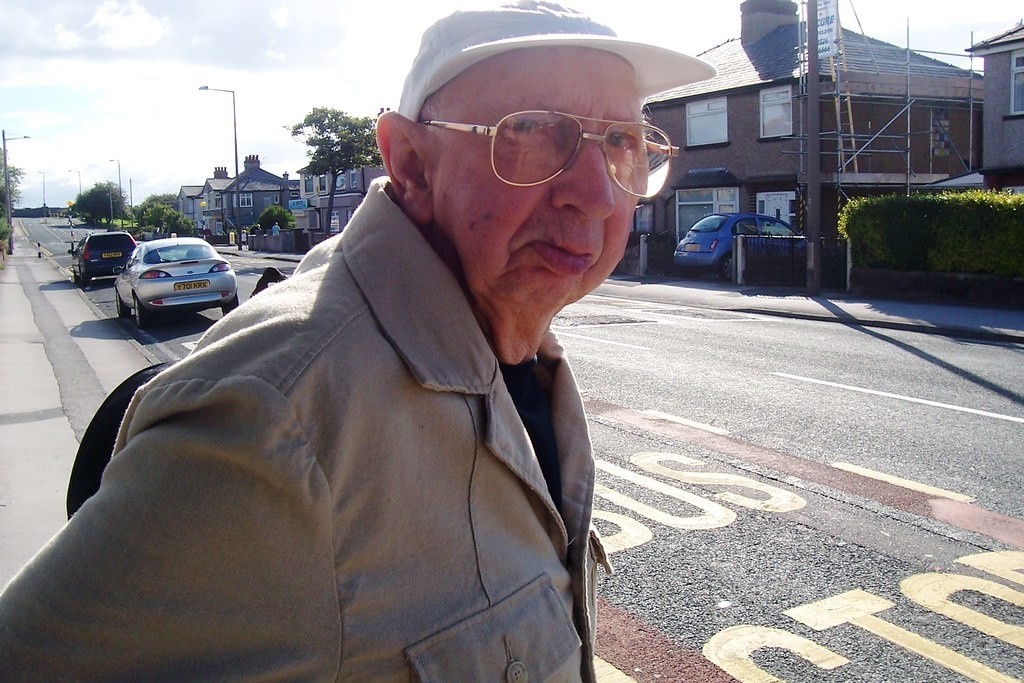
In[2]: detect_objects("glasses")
[422,110,682,198]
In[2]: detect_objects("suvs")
[68,229,138,287]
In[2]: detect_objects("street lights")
[109,159,124,228]
[69,169,82,194]
[38,171,46,217]
[2,130,32,256]
[199,86,243,251]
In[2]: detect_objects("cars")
[113,233,238,330]
[672,213,799,282]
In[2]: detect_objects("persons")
[254,223,262,230]
[0,0,720,683]
[241,229,247,249]
[272,222,281,236]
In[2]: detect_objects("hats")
[397,0,717,123]
